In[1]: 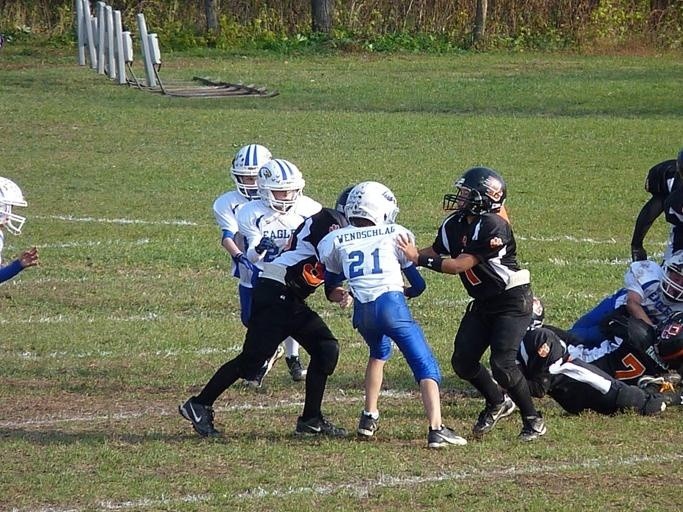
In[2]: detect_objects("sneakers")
[358,411,379,436]
[295,415,347,437]
[285,355,303,381]
[179,396,219,435]
[427,394,547,447]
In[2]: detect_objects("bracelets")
[418,254,445,273]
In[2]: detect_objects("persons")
[235,159,322,389]
[0,176,39,283]
[396,167,546,443]
[315,181,467,448]
[213,144,308,381]
[178,186,348,438]
[469,148,683,414]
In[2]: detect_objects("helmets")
[660,250,683,301]
[336,181,399,226]
[230,144,305,214]
[527,296,544,331]
[0,176,27,236]
[443,167,506,216]
